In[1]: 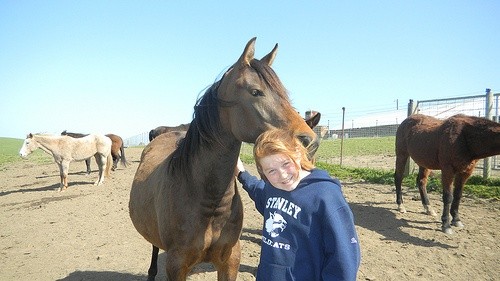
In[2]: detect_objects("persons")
[235,129,360,281]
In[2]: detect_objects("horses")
[394,113,500,235]
[128,36,321,281]
[19,130,131,192]
